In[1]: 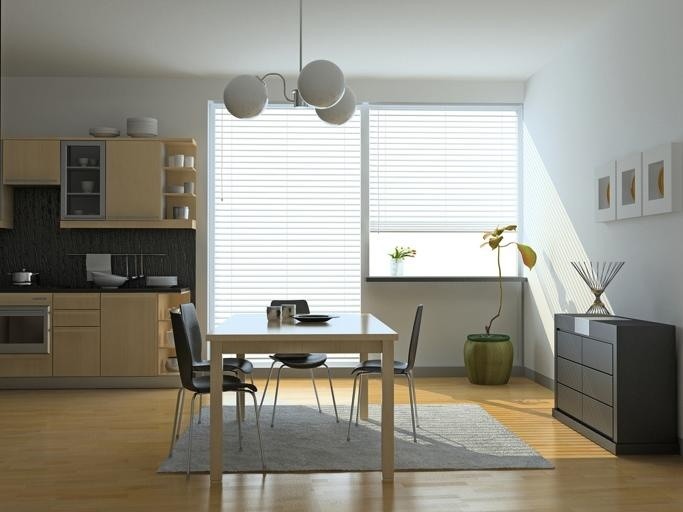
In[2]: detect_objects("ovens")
[0,304,52,355]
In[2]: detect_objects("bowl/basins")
[71,209,84,214]
[79,180,95,193]
[92,272,125,288]
[164,328,175,348]
[171,185,184,193]
[167,155,194,168]
[165,357,181,373]
[77,158,98,167]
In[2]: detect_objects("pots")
[8,269,39,285]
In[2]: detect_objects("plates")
[126,117,159,138]
[146,274,177,288]
[290,313,340,323]
[88,127,119,137]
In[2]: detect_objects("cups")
[184,181,194,193]
[265,306,281,322]
[281,304,295,322]
[173,206,189,218]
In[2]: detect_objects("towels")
[85,253,113,283]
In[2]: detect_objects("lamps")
[218,2,359,129]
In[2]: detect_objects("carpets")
[150,400,557,478]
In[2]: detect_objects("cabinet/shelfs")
[0,137,62,187]
[1,183,16,230]
[548,313,680,462]
[50,292,101,377]
[105,137,165,228]
[99,291,158,377]
[164,138,199,229]
[59,137,106,228]
[156,290,192,378]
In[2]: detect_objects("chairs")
[256,299,341,431]
[165,307,268,483]
[345,305,424,448]
[174,303,259,441]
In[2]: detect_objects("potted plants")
[383,244,416,276]
[461,223,536,387]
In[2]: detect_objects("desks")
[204,310,402,487]
